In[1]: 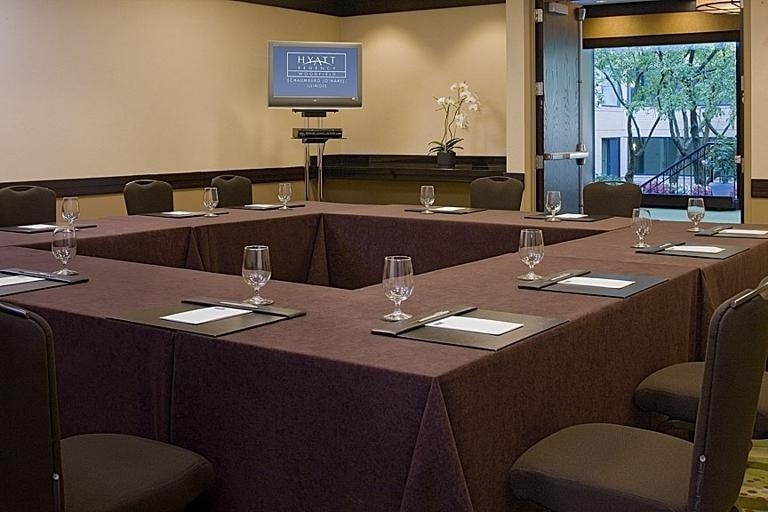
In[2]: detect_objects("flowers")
[429,81,481,153]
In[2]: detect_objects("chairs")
[124,179,174,215]
[468,176,527,211]
[212,172,253,207]
[0,184,57,224]
[584,182,643,220]
[509,280,768,511]
[1,304,215,511]
[634,277,768,438]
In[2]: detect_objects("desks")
[311,186,626,289]
[0,217,200,269]
[348,253,700,511]
[515,220,768,362]
[0,247,349,512]
[166,197,326,284]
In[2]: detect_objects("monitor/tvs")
[267,40,363,109]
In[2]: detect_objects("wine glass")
[60,196,80,232]
[687,197,706,232]
[420,186,435,213]
[51,227,81,276]
[277,183,293,210]
[240,244,275,306]
[544,191,563,222]
[516,228,544,281]
[202,187,219,217]
[381,254,415,322]
[630,207,652,248]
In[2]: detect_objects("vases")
[437,148,457,168]
